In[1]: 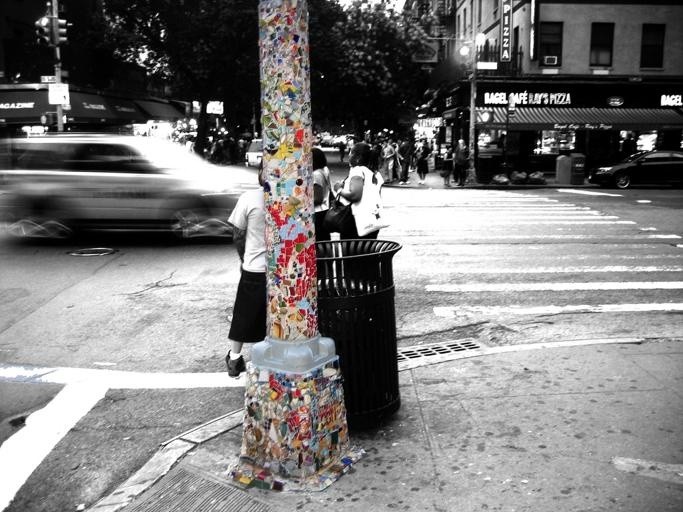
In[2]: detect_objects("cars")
[243,138,261,170]
[312,132,353,149]
[588,149,682,190]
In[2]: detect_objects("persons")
[226,160,266,376]
[339,141,346,162]
[337,142,383,255]
[312,148,331,241]
[171,121,252,165]
[622,134,637,157]
[444,138,468,188]
[436,122,446,155]
[348,136,433,185]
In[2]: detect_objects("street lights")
[458,30,488,187]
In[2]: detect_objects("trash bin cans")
[315,239,402,433]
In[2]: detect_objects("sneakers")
[398,181,464,188]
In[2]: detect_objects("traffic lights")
[57,19,69,46]
[34,20,40,47]
[37,16,51,49]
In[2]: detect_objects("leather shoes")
[225,349,246,377]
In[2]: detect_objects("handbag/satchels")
[322,192,357,237]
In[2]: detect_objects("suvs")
[0,131,261,247]
